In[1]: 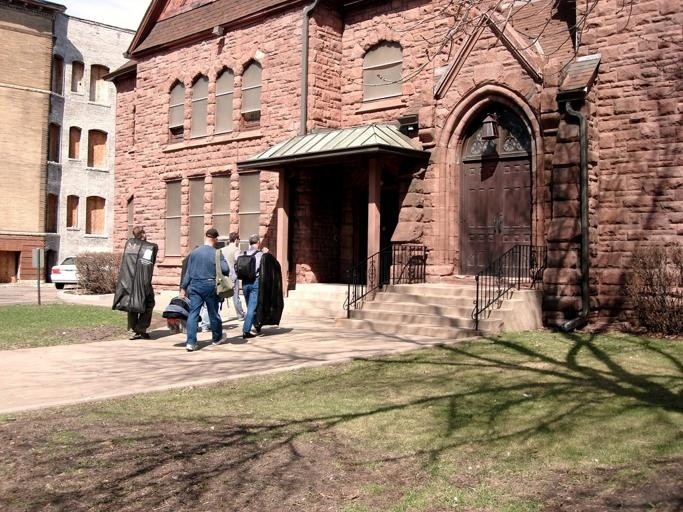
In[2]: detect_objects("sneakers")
[129,331,150,340]
[185,343,200,350]
[212,333,227,345]
[181,324,211,334]
[239,313,247,320]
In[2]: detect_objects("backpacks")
[235,251,260,283]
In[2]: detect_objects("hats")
[248,234,260,243]
[206,229,219,238]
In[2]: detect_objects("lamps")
[479,112,498,139]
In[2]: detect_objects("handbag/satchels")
[215,273,234,299]
[162,295,202,322]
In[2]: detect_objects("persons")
[241,235,270,339]
[178,228,230,351]
[179,246,212,334]
[216,232,247,323]
[126,225,155,340]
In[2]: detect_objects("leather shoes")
[255,325,262,333]
[243,332,255,339]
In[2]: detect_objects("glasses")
[237,238,241,241]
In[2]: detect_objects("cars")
[50,255,112,289]
